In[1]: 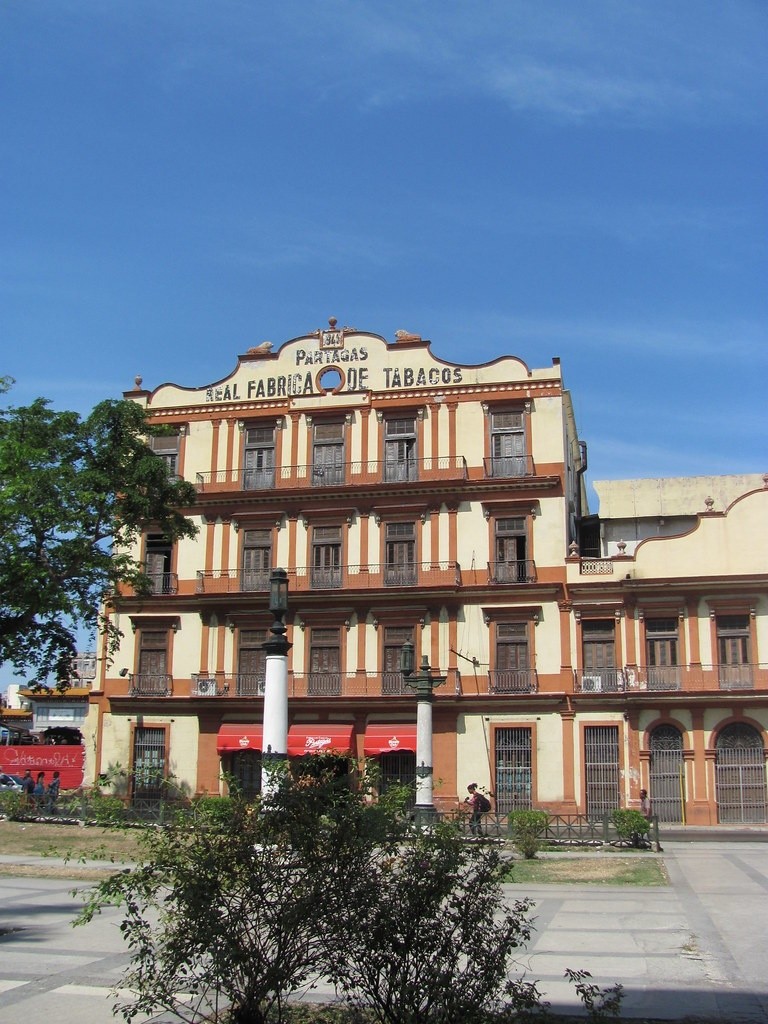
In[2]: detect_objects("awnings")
[365,725,416,756]
[217,723,263,751]
[287,725,358,756]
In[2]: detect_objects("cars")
[0,772,43,794]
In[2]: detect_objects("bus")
[0,722,84,791]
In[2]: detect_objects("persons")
[35,772,45,809]
[22,769,34,795]
[638,789,651,844]
[48,772,60,813]
[462,784,483,835]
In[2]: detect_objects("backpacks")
[474,794,491,812]
